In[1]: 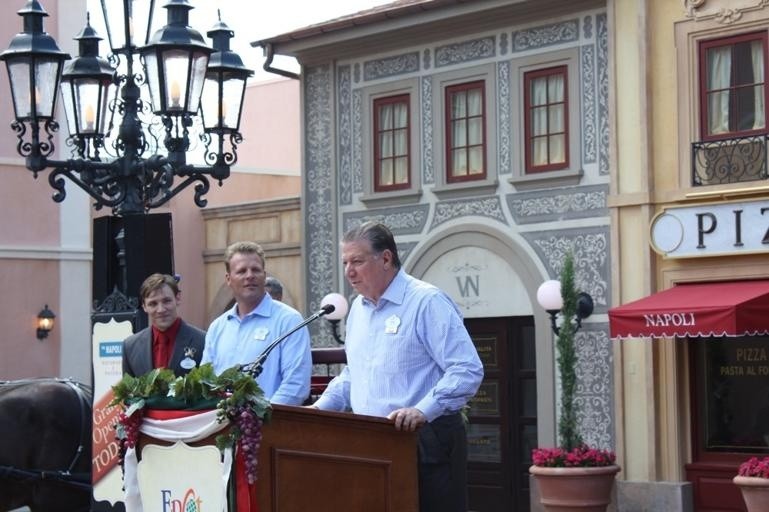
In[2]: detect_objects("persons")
[264,277,283,302]
[297,221,486,512]
[197,241,312,406]
[120,274,207,381]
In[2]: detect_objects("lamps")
[537,280,592,338]
[37,304,56,340]
[319,293,348,344]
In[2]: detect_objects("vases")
[733,476,769,512]
[529,466,621,512]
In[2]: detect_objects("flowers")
[738,458,769,477]
[529,247,617,465]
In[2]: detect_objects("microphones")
[248,304,335,379]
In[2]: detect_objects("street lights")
[0,0,255,337]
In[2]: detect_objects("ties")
[156,335,170,369]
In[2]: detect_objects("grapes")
[112,408,144,491]
[216,397,264,485]
